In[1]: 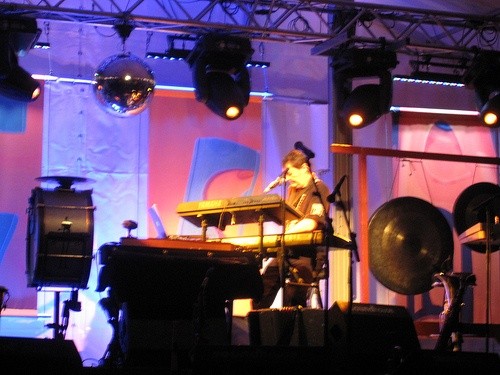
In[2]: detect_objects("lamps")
[187,33,255,121]
[461,46,500,127]
[0,15,42,103]
[329,37,398,129]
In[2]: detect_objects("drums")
[25,186,95,288]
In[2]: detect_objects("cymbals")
[33,175,97,185]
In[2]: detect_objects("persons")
[249,150,330,310]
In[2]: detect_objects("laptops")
[148,207,201,241]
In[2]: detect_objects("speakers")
[0,336,85,375]
[246,301,421,352]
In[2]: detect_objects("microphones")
[294,141,315,158]
[327,174,346,203]
[264,178,285,193]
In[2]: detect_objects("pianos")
[176,193,352,309]
